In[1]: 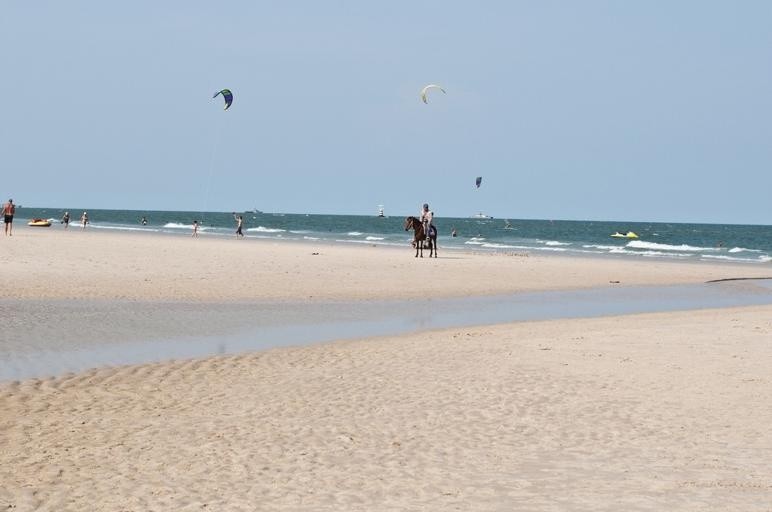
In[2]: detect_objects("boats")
[610,231,638,238]
[27,218,50,226]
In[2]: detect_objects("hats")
[423,204,428,208]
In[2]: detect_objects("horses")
[405,215,439,258]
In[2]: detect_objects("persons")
[419,203,433,241]
[141,217,148,225]
[233,214,244,237]
[61,212,72,228]
[452,230,457,238]
[32,217,37,222]
[80,211,89,228]
[191,220,198,238]
[0,198,15,236]
